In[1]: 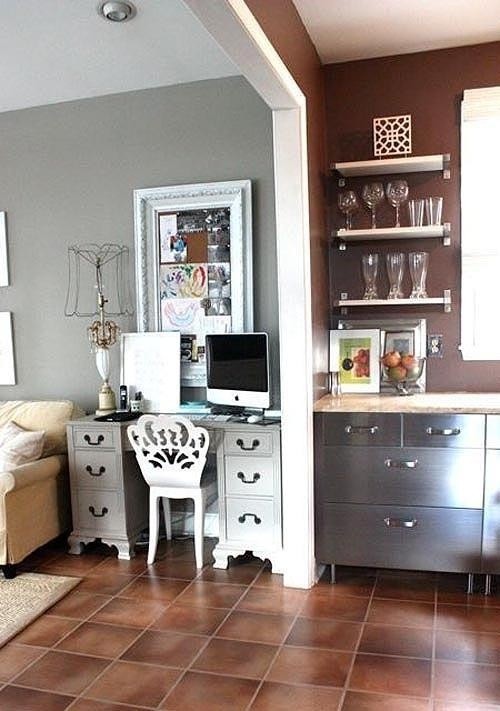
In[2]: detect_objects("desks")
[62,413,283,575]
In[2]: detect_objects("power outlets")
[427,334,443,357]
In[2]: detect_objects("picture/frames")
[133,180,253,387]
[337,317,426,391]
[329,328,381,394]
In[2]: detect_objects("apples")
[380,350,423,380]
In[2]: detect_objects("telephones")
[115,385,131,414]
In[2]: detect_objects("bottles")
[119,384,128,410]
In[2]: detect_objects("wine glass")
[338,179,410,230]
[379,358,425,396]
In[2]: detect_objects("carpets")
[0,572,82,648]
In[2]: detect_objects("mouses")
[247,414,263,424]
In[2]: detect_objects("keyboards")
[159,415,233,422]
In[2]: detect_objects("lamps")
[62,241,133,416]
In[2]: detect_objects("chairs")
[126,414,219,568]
[0,399,87,578]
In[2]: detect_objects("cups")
[130,400,141,412]
[407,198,424,228]
[360,251,430,299]
[326,371,342,396]
[426,196,443,227]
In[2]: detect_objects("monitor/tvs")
[205,333,270,416]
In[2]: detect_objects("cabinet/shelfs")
[315,413,486,595]
[481,412,500,594]
[331,153,452,315]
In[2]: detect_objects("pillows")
[0,421,46,474]
